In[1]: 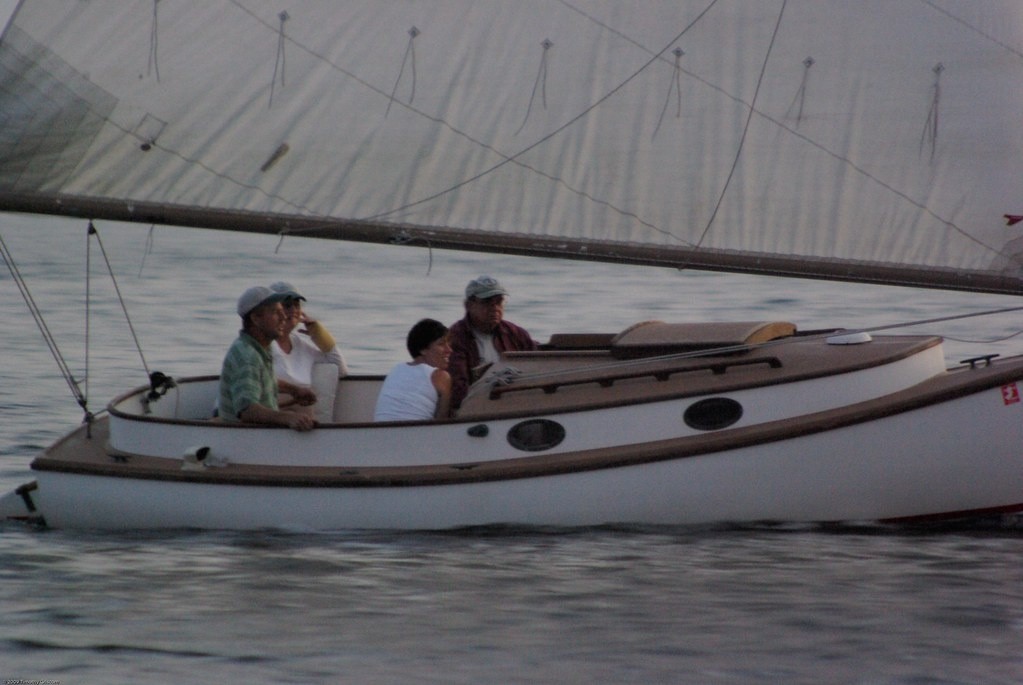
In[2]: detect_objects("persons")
[269,281,349,407]
[446,274,538,410]
[373,318,453,424]
[216,285,318,432]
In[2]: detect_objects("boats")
[0,317,1023,546]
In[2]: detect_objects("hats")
[236,285,290,316]
[464,275,510,301]
[408,318,448,359]
[269,283,307,304]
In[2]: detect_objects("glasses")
[473,298,504,306]
